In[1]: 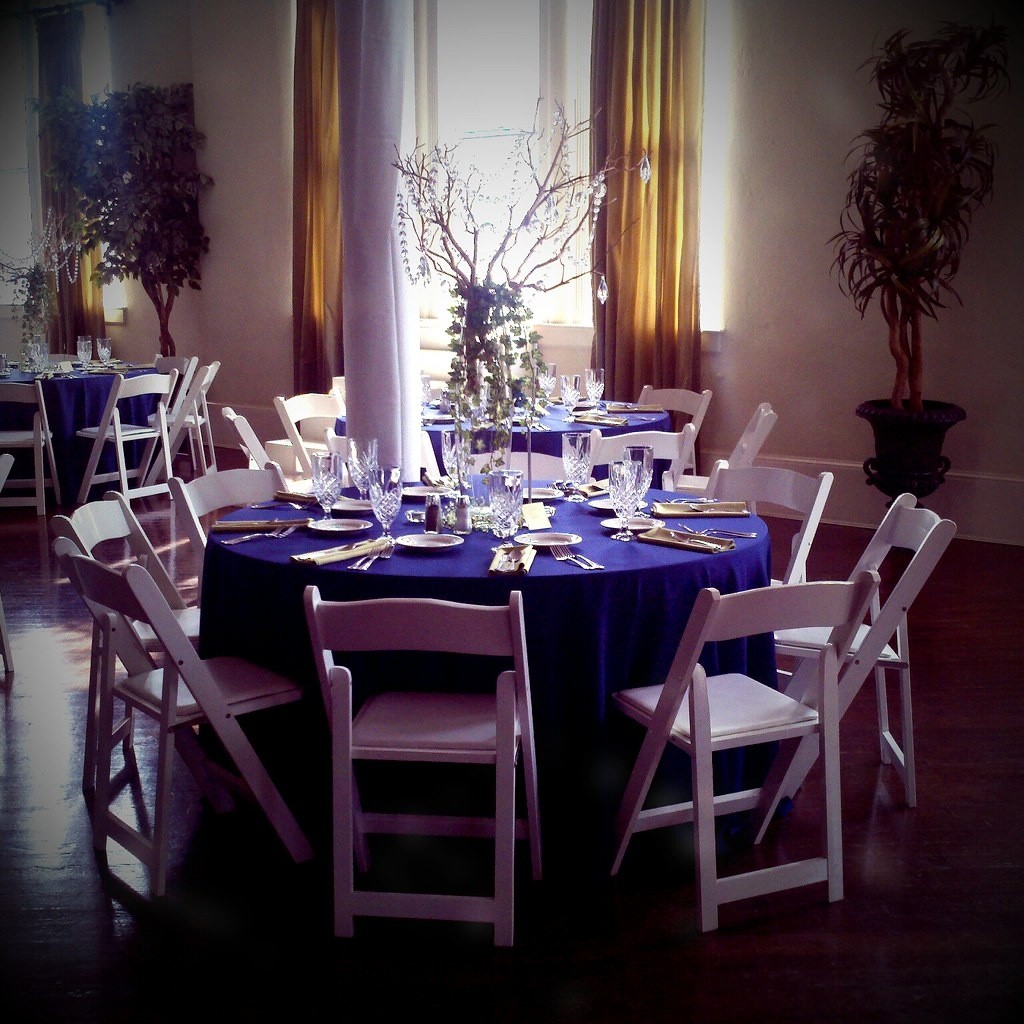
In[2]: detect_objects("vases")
[454,382,515,531]
[19,298,48,372]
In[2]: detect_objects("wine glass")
[77,335,112,374]
[623,445,654,518]
[368,464,403,545]
[346,436,379,501]
[489,469,524,554]
[585,368,605,414]
[608,460,642,542]
[310,451,343,521]
[536,363,556,406]
[421,375,430,419]
[562,433,592,503]
[561,375,581,422]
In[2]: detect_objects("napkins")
[487,543,538,577]
[578,478,609,498]
[636,526,736,554]
[422,472,456,491]
[650,501,752,518]
[290,536,393,566]
[606,403,665,414]
[575,414,629,427]
[211,518,315,532]
[273,490,355,503]
[421,414,456,424]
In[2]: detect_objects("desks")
[0,362,162,506]
[185,479,795,944]
[334,399,672,492]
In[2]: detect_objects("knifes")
[495,552,509,571]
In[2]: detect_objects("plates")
[332,500,374,515]
[514,533,582,546]
[396,533,464,553]
[523,488,564,499]
[424,414,456,424]
[588,498,649,509]
[600,517,666,530]
[307,519,373,535]
[401,486,454,500]
[45,371,70,375]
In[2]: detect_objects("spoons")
[507,549,523,570]
[689,505,748,513]
[670,532,722,548]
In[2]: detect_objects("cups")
[441,429,471,485]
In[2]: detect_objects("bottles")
[0,354,6,373]
[453,496,472,535]
[424,494,444,534]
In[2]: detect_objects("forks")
[652,497,715,504]
[221,523,302,545]
[549,545,595,569]
[677,523,758,538]
[251,500,318,510]
[357,545,394,570]
[347,545,382,569]
[558,545,605,569]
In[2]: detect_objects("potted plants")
[826,7,1012,509]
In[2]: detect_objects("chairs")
[0,354,958,949]
